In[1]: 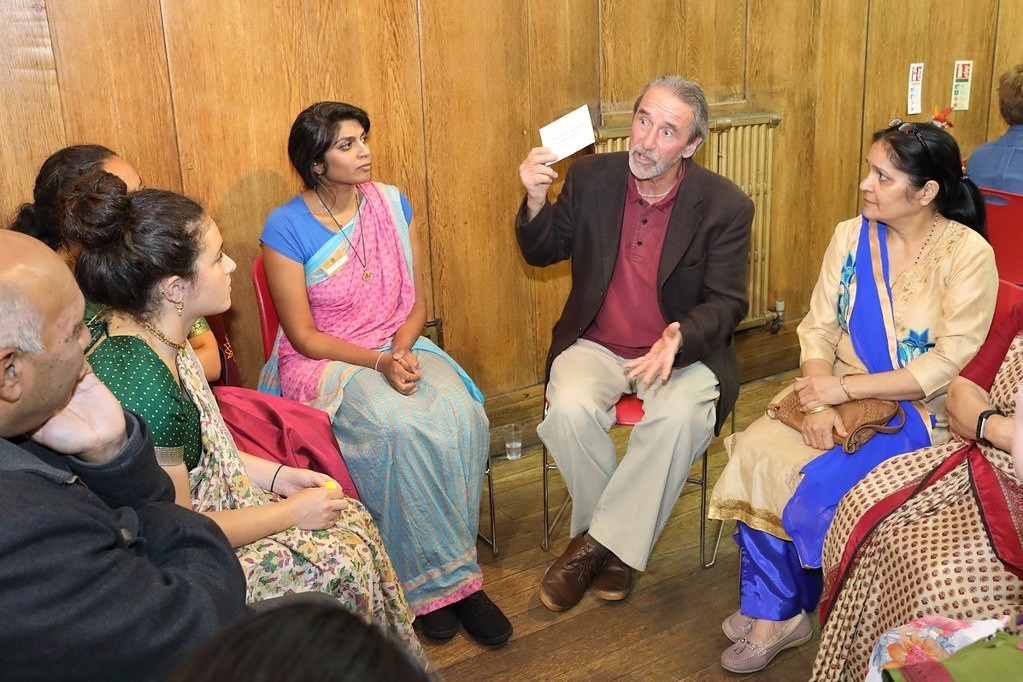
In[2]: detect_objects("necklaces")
[914,208,940,266]
[125,308,186,350]
[635,190,671,199]
[314,180,371,282]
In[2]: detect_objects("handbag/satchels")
[765,390,905,454]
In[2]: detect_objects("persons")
[721,121,1001,674]
[259,102,516,646]
[514,74,758,613]
[0,141,433,681]
[965,63,1023,203]
[819,302,1023,656]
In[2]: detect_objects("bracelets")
[840,373,857,402]
[270,464,284,492]
[374,352,386,371]
[804,404,833,415]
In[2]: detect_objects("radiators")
[591,112,775,332]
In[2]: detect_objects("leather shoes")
[591,551,633,600]
[539,529,606,611]
[721,608,813,673]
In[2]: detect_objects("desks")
[882,616,1023,682]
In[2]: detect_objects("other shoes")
[421,604,458,638]
[455,590,513,646]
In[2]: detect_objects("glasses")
[888,118,937,181]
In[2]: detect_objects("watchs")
[976,410,1005,447]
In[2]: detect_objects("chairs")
[541,389,735,568]
[976,188,1023,288]
[253,253,498,559]
[958,279,1023,393]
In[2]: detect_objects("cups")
[502,424,523,460]
